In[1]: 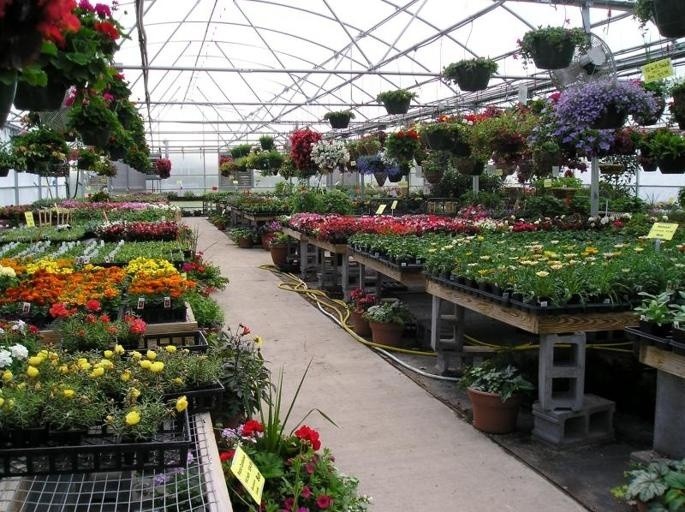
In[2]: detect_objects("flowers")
[0,0,174,178]
